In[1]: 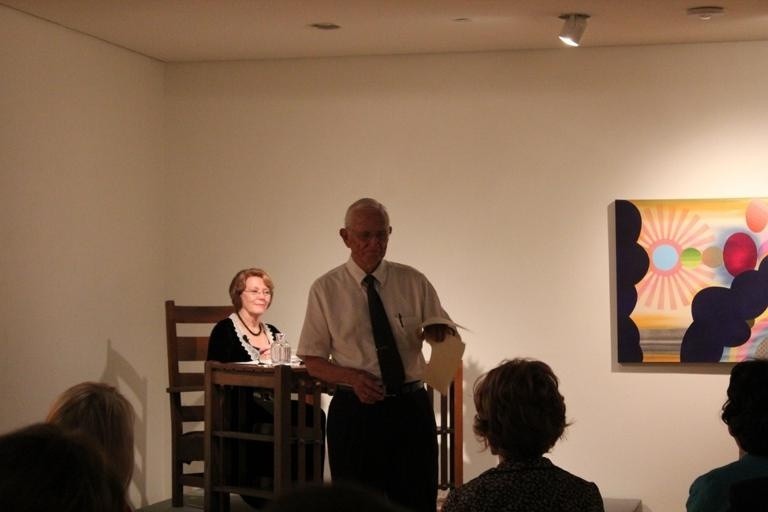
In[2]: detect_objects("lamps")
[557,13,590,47]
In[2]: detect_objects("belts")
[382,381,424,397]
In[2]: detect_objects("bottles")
[271,331,291,364]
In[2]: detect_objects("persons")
[433,355,604,512]
[41,380,137,504]
[295,196,458,512]
[682,358,765,511]
[201,267,326,505]
[0,422,134,510]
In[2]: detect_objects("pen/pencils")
[398,313,404,328]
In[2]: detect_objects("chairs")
[165,300,234,512]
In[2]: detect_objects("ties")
[363,275,405,395]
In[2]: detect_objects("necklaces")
[235,310,262,337]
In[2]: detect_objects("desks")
[202,361,462,512]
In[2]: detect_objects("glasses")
[349,227,390,241]
